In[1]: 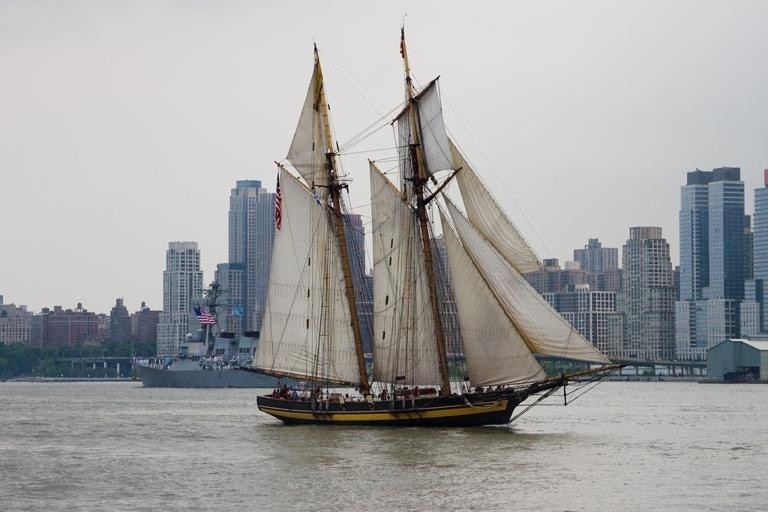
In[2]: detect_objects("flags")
[192,306,216,325]
[275,173,282,230]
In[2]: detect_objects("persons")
[272,384,309,401]
[462,385,494,393]
[316,386,321,399]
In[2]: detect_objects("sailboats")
[253,17,634,430]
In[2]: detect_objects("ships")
[131,282,317,389]
